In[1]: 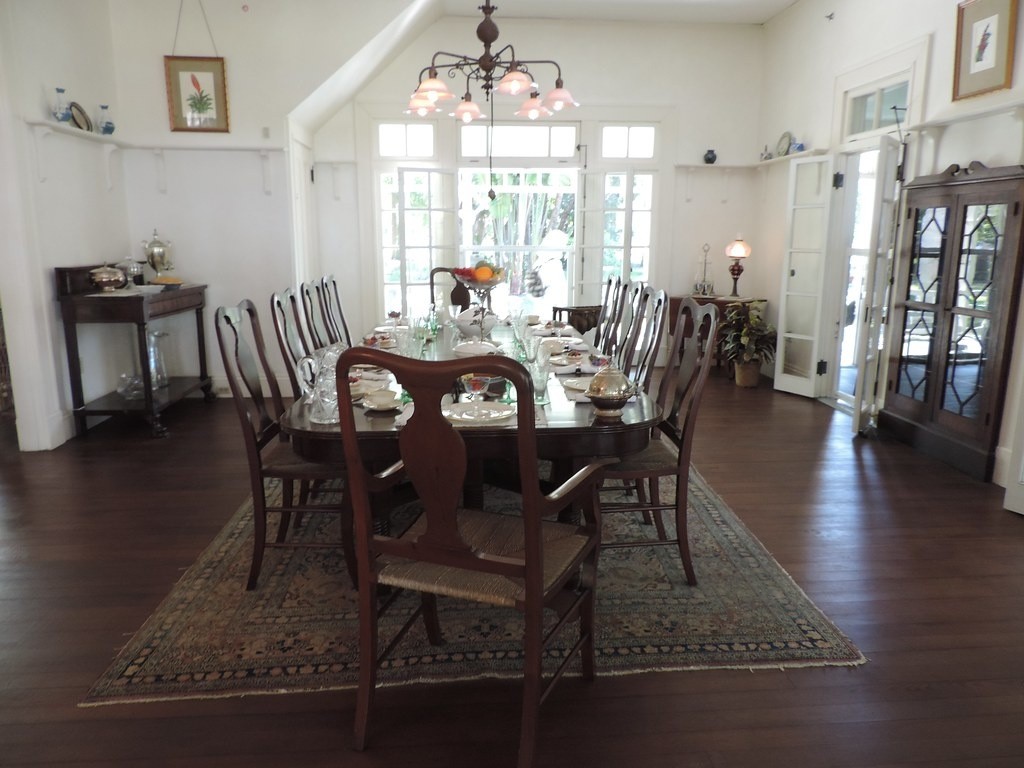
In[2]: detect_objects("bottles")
[704,150,716,164]
[116,371,157,401]
[98,106,115,135]
[51,88,73,121]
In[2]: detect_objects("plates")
[439,402,515,422]
[71,102,93,132]
[776,132,791,156]
[529,322,636,392]
[340,326,410,410]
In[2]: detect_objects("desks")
[279,313,663,590]
[669,294,766,369]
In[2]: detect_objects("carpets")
[80,419,866,709]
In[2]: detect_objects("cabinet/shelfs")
[53,261,211,436]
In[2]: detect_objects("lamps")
[406,2,580,204]
[725,240,751,297]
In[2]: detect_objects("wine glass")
[394,303,552,405]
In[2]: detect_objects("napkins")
[533,328,572,336]
[556,364,608,375]
[565,343,589,351]
[349,371,385,381]
[575,393,636,404]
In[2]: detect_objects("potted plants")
[720,300,777,385]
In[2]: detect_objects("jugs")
[295,341,349,424]
[145,332,171,388]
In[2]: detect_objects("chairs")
[214,273,718,768]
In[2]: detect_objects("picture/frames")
[165,56,230,133]
[951,0,1017,100]
